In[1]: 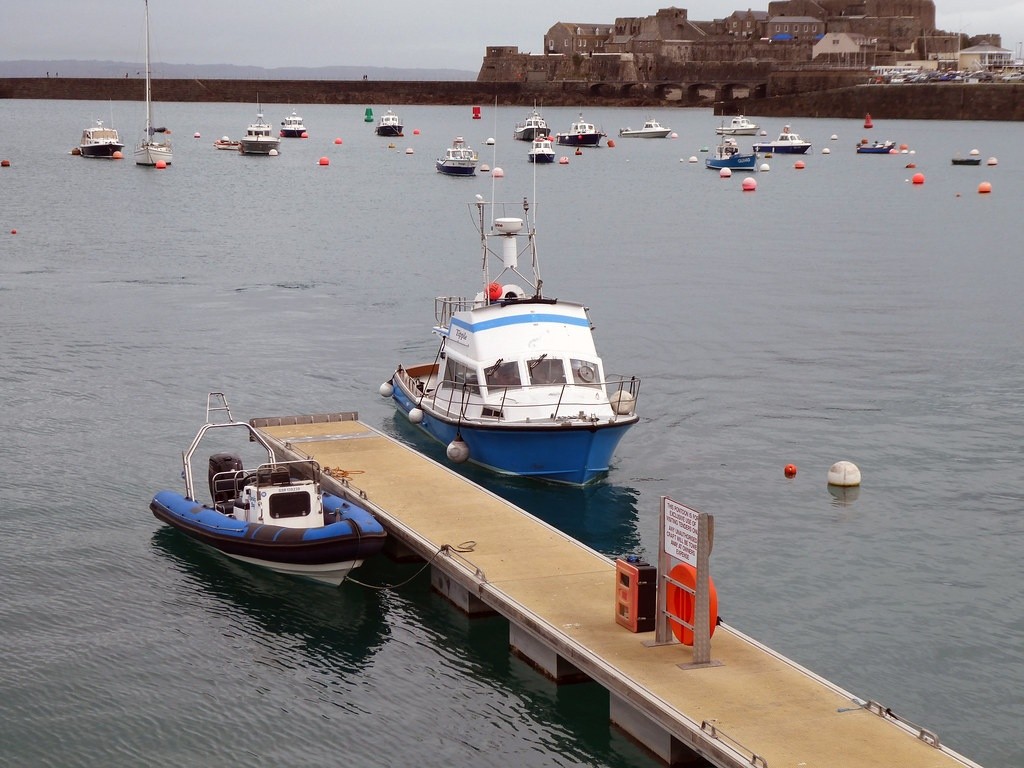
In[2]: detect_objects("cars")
[868,66,1024,84]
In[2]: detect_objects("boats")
[526,131,556,165]
[435,135,480,177]
[214,137,241,150]
[238,99,282,156]
[704,118,758,171]
[148,390,388,587]
[714,111,760,136]
[951,158,983,165]
[618,117,673,139]
[554,104,607,148]
[751,124,813,154]
[374,106,405,136]
[278,109,307,138]
[513,96,552,143]
[380,92,644,488]
[77,120,125,160]
[854,140,896,154]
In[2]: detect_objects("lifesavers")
[496,284,526,301]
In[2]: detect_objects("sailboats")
[132,0,174,167]
